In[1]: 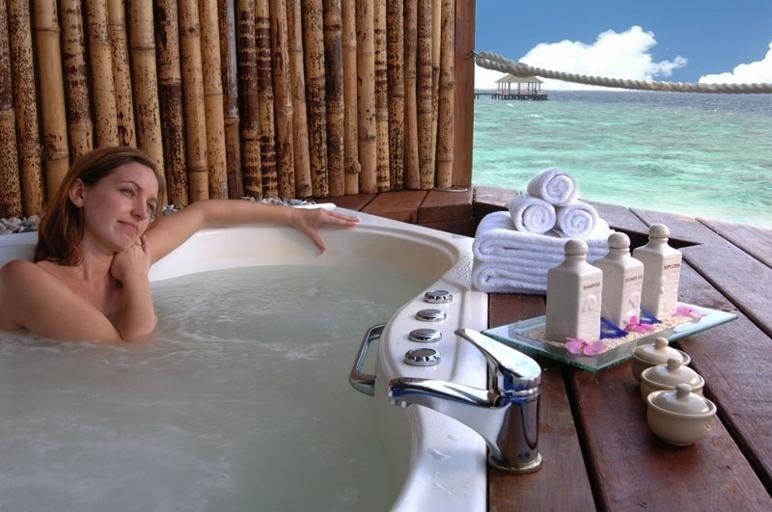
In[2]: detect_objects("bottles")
[544,223,683,345]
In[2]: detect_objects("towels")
[509,194,556,235]
[552,201,598,238]
[472,211,615,269]
[527,169,578,207]
[472,258,548,295]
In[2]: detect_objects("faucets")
[385,325,549,472]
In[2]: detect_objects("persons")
[0,145,361,348]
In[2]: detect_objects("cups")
[631,337,718,447]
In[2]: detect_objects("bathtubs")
[0,205,490,512]
